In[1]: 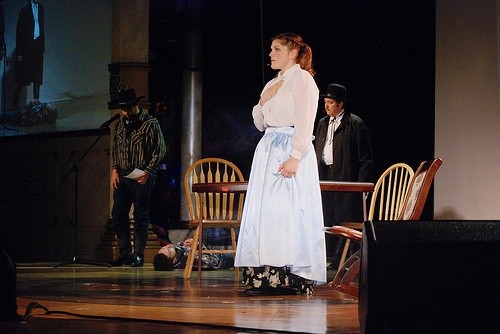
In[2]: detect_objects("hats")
[320,83,353,102]
[107,88,146,108]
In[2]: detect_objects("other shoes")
[246,286,278,295]
[131,255,144,266]
[110,257,130,266]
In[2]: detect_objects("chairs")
[324,157,442,297]
[182,158,245,279]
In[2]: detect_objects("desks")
[192,181,375,283]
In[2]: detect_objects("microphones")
[101,113,120,128]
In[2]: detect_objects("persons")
[108,88,165,267]
[153,238,234,271]
[315,84,371,270]
[235,32,327,297]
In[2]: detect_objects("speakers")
[358,220,500,334]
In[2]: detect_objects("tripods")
[55,126,112,269]
